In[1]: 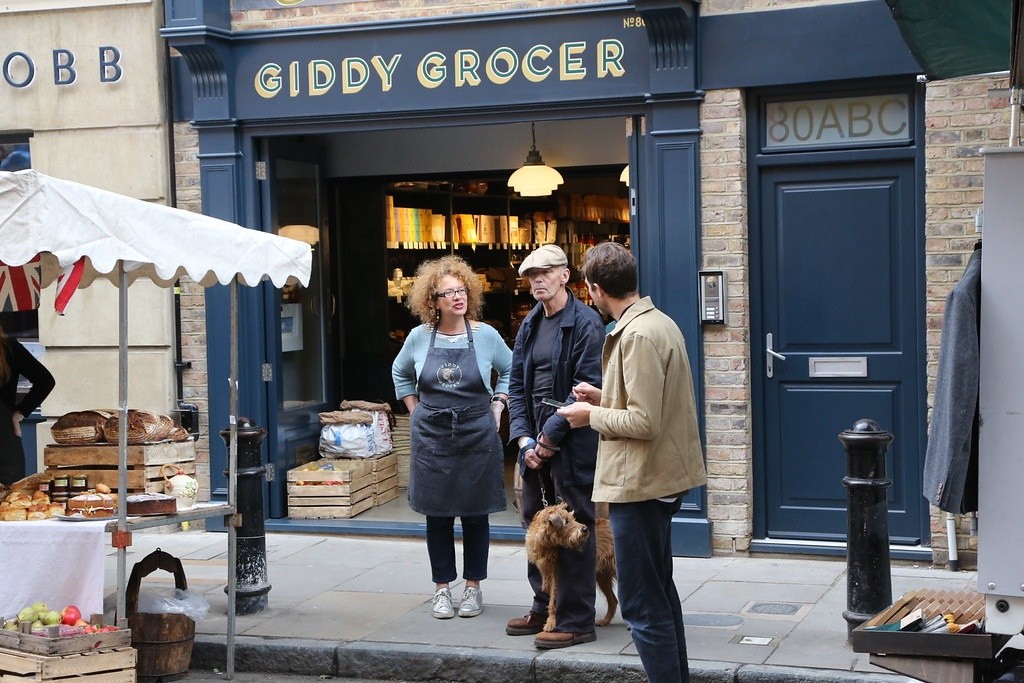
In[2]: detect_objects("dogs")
[524,501,618,633]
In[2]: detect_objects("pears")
[3,603,61,632]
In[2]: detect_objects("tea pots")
[162,464,199,508]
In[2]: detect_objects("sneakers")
[432,588,454,618]
[458,587,482,618]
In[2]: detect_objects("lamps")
[506,121,565,197]
[619,164,630,188]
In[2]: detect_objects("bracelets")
[490,396,507,408]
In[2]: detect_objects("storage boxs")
[287,414,411,520]
[43,437,195,502]
[0,646,139,682]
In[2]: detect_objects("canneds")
[38,474,88,515]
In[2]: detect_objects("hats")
[518,244,568,277]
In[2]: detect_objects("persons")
[504,246,606,647]
[391,254,513,618]
[0,334,56,485]
[557,243,709,683]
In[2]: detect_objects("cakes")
[124,492,178,514]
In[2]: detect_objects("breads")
[67,483,118,518]
[0,471,66,521]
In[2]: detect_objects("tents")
[0,168,313,681]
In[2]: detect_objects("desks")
[0,502,242,681]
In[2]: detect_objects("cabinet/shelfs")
[326,172,631,415]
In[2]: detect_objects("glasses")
[436,287,469,298]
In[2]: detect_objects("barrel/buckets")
[125,548,195,683]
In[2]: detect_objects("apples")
[60,605,82,626]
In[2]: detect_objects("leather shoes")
[534,631,597,648]
[506,611,546,636]
